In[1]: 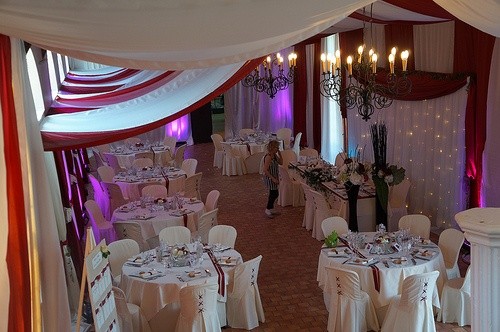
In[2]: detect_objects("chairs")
[85,128,471,332]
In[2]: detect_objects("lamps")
[318,2,411,121]
[241,51,298,99]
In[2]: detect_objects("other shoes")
[271,210,281,215]
[264,212,274,219]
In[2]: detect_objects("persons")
[262,141,283,217]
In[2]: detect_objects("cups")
[118,164,186,185]
[115,192,205,221]
[224,132,280,146]
[322,224,440,270]
[103,139,170,156]
[123,235,242,283]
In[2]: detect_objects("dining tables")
[111,197,205,252]
[113,166,187,203]
[317,232,448,329]
[221,136,283,176]
[122,242,243,332]
[291,175,389,241]
[103,145,171,172]
